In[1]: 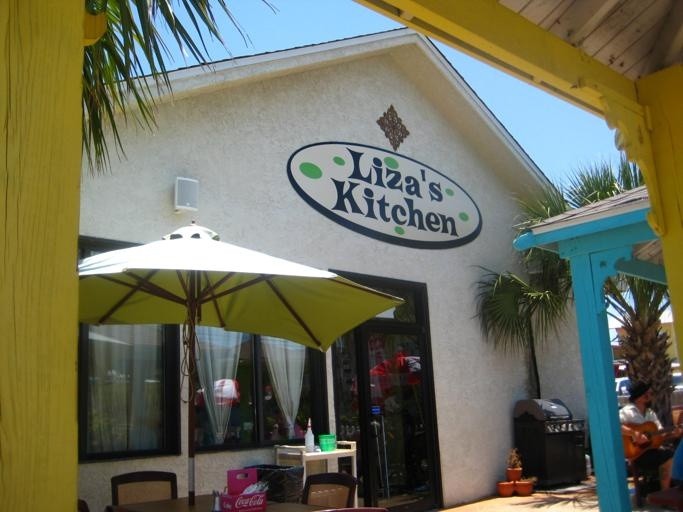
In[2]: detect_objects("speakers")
[173,177,200,212]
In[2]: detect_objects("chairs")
[301,472,358,509]
[106,470,177,509]
[245,464,304,503]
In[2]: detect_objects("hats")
[627,380,653,403]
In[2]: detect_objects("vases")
[494,467,534,496]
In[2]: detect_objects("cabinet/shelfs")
[274,440,358,508]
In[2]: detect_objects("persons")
[617,380,673,490]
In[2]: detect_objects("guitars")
[620,420,683,461]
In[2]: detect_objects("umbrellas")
[194,379,241,432]
[349,354,425,426]
[79,219,405,508]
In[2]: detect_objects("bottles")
[304,416,315,453]
